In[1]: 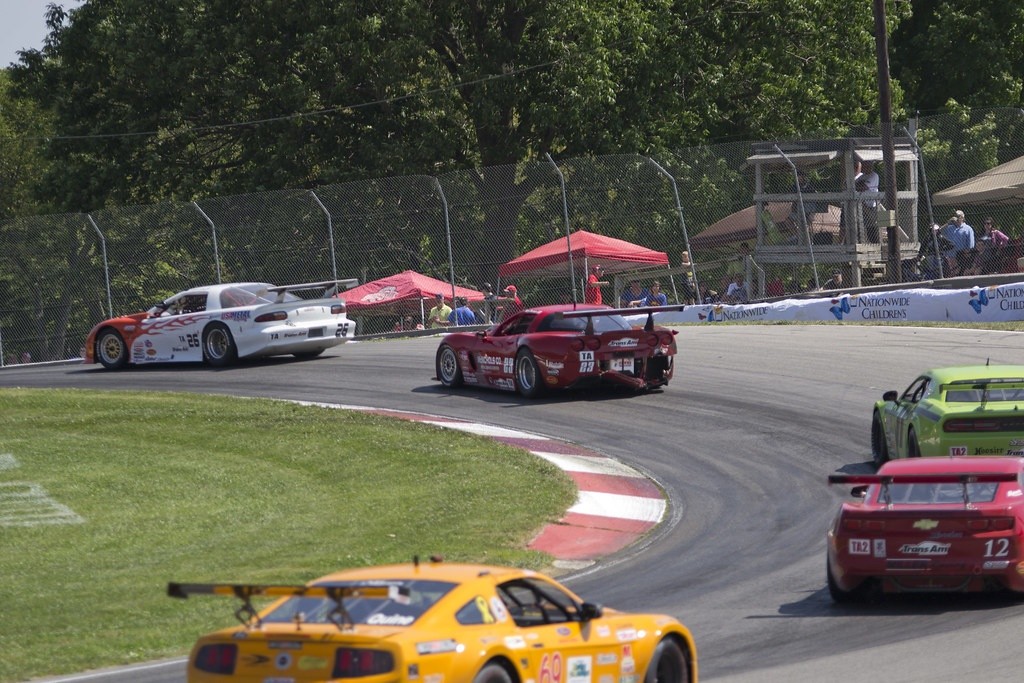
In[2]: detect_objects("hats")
[502,285,517,292]
[478,282,493,292]
[930,224,940,230]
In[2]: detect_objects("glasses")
[984,222,991,225]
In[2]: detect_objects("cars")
[80,282,358,369]
[167,555,699,683]
[871,358,1024,467]
[827,456,1024,605]
[431,304,685,399]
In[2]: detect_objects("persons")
[185,295,207,313]
[496,285,524,324]
[787,161,880,244]
[913,209,1009,281]
[428,294,453,329]
[696,270,842,304]
[449,297,475,326]
[627,281,667,308]
[481,282,495,324]
[394,315,416,332]
[610,279,650,308]
[585,264,609,305]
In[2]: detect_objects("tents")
[495,229,682,326]
[931,154,1024,206]
[331,270,507,329]
[688,202,910,244]
